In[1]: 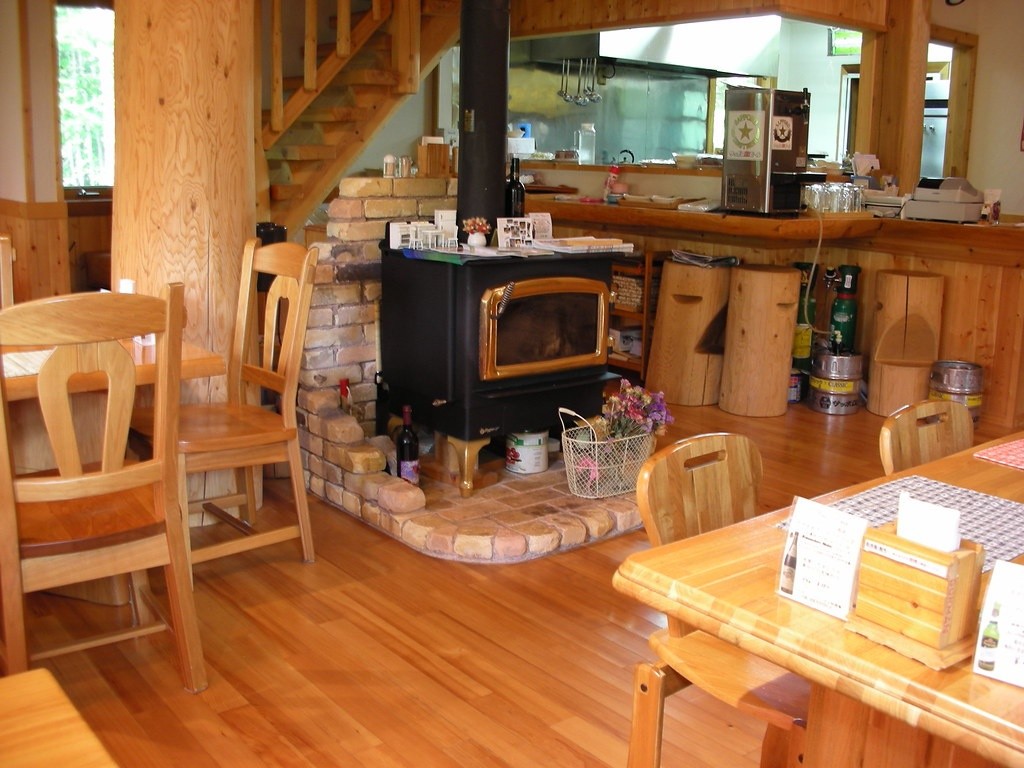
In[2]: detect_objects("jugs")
[573,122,597,167]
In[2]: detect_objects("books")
[497,236,633,258]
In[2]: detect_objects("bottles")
[778,530,800,595]
[395,404,421,486]
[505,157,525,218]
[976,605,1000,672]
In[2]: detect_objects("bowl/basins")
[672,155,696,170]
[556,150,575,159]
[825,167,846,177]
[808,165,827,173]
[508,130,526,138]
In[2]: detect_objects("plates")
[650,194,680,205]
[639,159,676,169]
[622,193,651,202]
[552,159,577,166]
[526,158,553,164]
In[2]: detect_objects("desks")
[3,333,230,606]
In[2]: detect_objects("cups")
[517,122,531,139]
[607,194,624,204]
[398,155,413,178]
[382,153,397,179]
[803,180,866,215]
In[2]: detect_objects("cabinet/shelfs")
[607,252,663,381]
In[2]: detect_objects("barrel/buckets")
[504,426,550,475]
[806,347,866,416]
[925,360,985,431]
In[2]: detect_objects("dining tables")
[612,427,1023,768]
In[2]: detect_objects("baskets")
[558,407,657,499]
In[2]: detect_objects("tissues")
[842,489,986,673]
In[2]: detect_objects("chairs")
[123,236,320,594]
[877,397,975,476]
[626,430,814,768]
[1,279,210,696]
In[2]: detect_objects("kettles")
[610,149,635,166]
